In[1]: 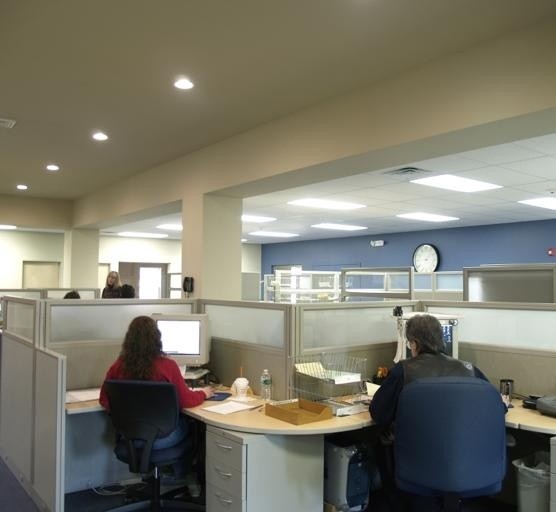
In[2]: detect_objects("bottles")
[259,369,271,400]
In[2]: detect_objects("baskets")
[288,351,367,416]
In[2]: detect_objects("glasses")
[406,339,411,350]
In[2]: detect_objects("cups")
[500,378,514,404]
[234,378,250,397]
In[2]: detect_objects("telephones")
[183,276,193,292]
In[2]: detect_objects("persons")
[118,284,135,298]
[96,315,220,501]
[100,269,121,298]
[365,314,508,511]
[60,290,81,299]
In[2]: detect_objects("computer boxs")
[324,440,370,512]
[182,368,211,389]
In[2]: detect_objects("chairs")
[105,380,206,512]
[393,378,507,512]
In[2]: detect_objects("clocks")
[412,243,439,272]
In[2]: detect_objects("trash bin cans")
[512,451,550,511]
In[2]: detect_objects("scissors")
[377,367,388,379]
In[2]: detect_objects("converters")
[120,477,142,486]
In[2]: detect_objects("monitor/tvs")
[397,312,458,361]
[151,313,211,373]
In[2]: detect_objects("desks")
[65,380,556,436]
